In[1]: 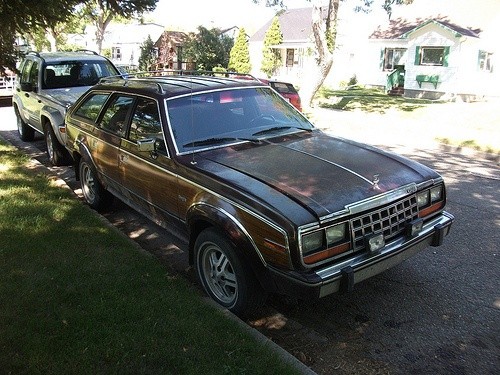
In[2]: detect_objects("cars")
[233,74,303,114]
[63,71,457,312]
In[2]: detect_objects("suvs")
[12,49,130,167]
